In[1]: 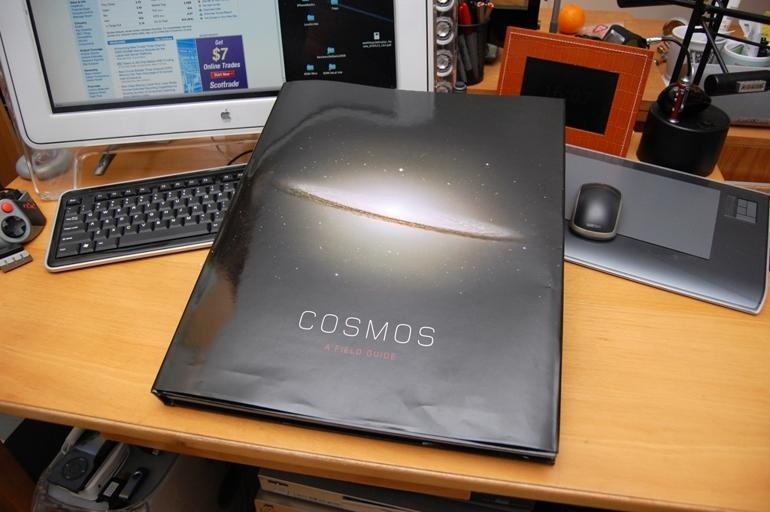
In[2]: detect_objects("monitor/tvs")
[0,0,435,202]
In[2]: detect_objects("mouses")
[569,182,624,242]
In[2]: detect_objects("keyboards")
[44,162,249,274]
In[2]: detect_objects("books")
[254,466,621,511]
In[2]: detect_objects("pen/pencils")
[454,0,495,86]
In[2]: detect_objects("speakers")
[433,0,458,94]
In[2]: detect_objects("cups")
[459,23,486,85]
[666,25,726,82]
[724,43,769,68]
